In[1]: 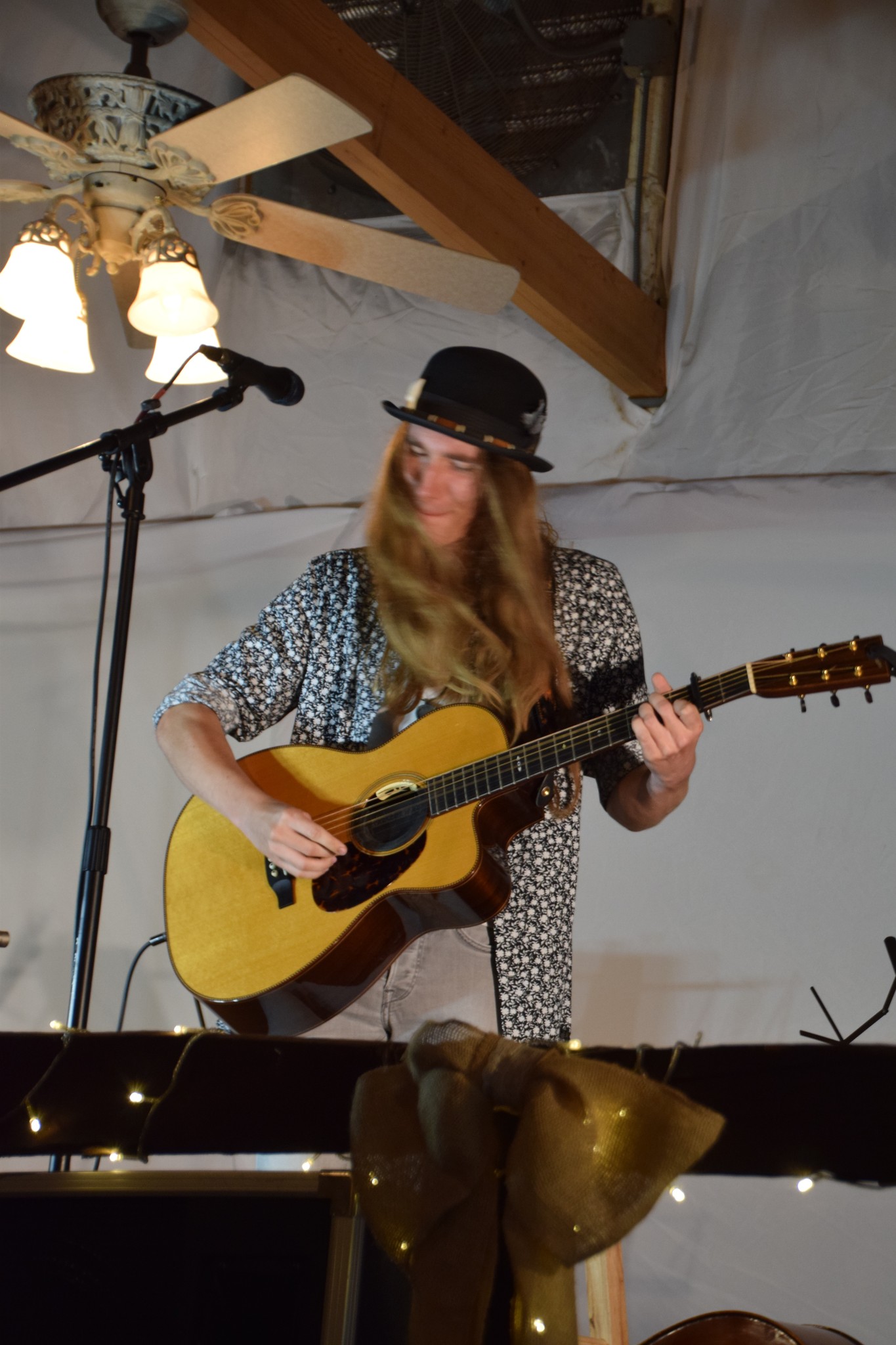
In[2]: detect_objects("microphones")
[200,344,304,407]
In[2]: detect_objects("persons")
[151,344,705,1043]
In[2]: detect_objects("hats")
[380,345,554,474]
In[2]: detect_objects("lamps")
[0,203,232,387]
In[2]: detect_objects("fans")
[0,0,522,321]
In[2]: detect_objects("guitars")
[161,627,895,1034]
